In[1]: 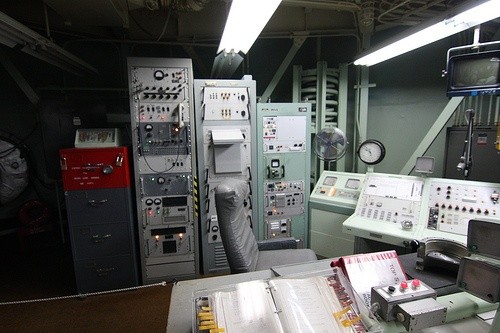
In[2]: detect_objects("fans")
[312,127,349,171]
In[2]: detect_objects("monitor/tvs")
[447,41,500,96]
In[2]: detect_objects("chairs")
[212,175,319,276]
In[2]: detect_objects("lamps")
[0,12,48,49]
[214,0,281,55]
[352,0,500,68]
[208,52,244,79]
[20,40,98,80]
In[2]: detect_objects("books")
[207,276,358,333]
